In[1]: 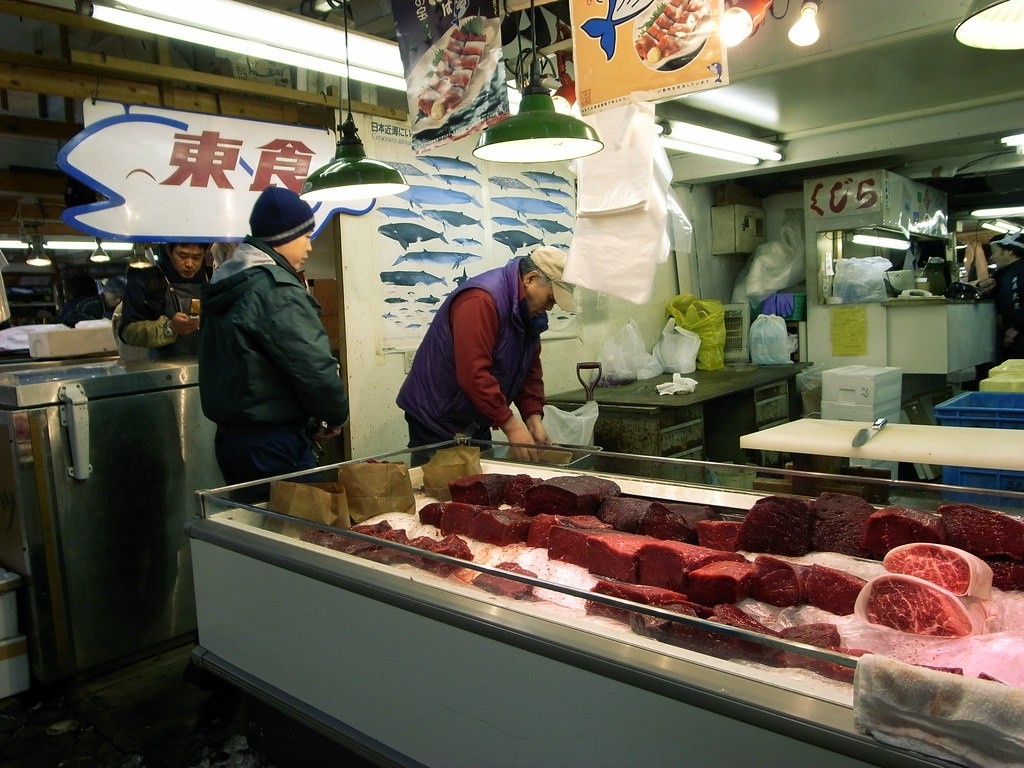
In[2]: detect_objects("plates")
[408,16,501,135]
[633,0,711,72]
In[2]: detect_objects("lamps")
[74,0,409,92]
[970,205,1024,235]
[717,0,772,48]
[954,0,1024,51]
[300,0,408,204]
[647,121,784,166]
[843,232,911,252]
[473,1,607,166]
[552,55,578,114]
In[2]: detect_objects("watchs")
[164,319,176,337]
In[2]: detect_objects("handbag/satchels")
[832,257,892,302]
[590,298,793,387]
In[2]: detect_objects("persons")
[75,279,124,319]
[395,247,578,468]
[118,242,211,359]
[988,234,1024,362]
[112,301,149,359]
[196,187,349,501]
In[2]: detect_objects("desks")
[545,359,814,462]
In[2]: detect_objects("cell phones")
[186,298,200,319]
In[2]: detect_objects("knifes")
[852,417,887,447]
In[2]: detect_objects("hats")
[530,246,578,313]
[251,186,315,247]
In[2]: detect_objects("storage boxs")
[27,324,118,359]
[821,349,1024,501]
